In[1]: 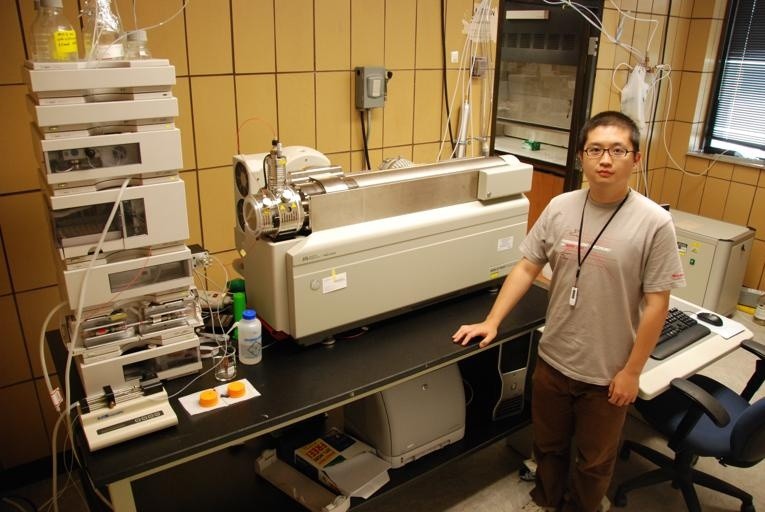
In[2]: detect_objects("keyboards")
[650,307,710,361]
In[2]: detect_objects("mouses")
[697,313,723,326]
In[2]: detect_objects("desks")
[45,281,552,512]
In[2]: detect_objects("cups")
[212,344,237,380]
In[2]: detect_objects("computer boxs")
[483,331,534,422]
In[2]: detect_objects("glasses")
[582,144,638,159]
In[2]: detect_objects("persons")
[451,109,687,511]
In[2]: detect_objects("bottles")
[238,311,263,365]
[31,0,78,62]
[85,1,152,60]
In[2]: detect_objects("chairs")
[610,339,764,510]
